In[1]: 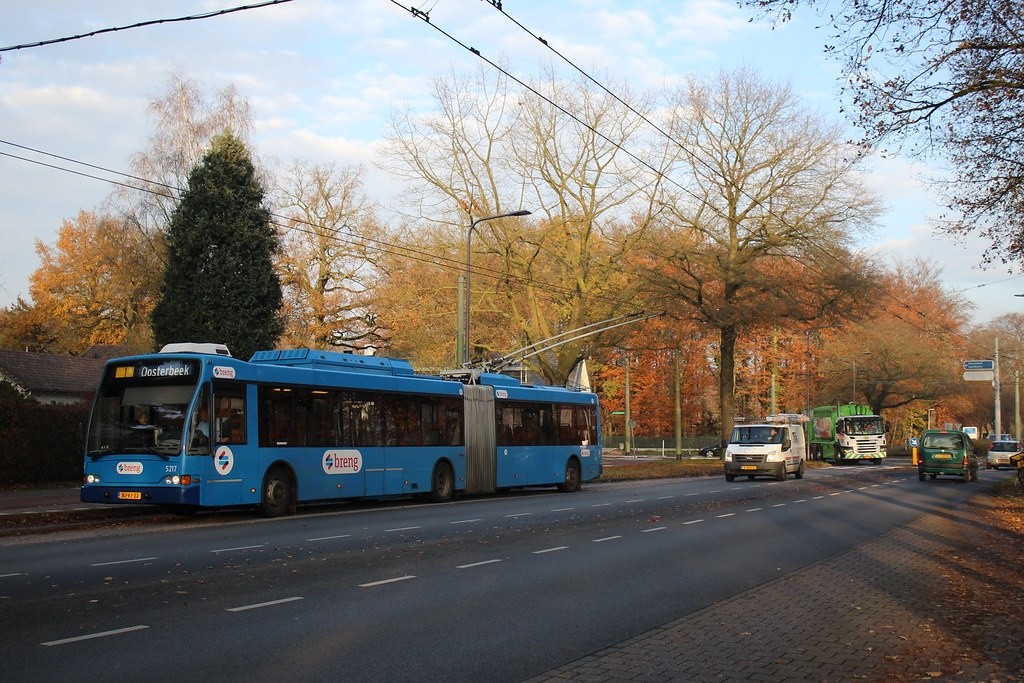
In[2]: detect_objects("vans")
[917,430,981,483]
[721,422,807,482]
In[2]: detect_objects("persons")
[768,429,777,440]
[866,422,877,431]
[196,407,220,441]
[136,409,148,426]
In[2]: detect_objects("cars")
[698,441,721,458]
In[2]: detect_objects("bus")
[79,310,666,520]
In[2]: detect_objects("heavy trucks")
[805,402,891,466]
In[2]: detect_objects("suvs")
[986,440,1024,470]
[985,434,1013,441]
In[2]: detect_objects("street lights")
[806,324,844,461]
[853,352,872,403]
[464,209,533,366]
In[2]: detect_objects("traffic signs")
[962,360,993,370]
